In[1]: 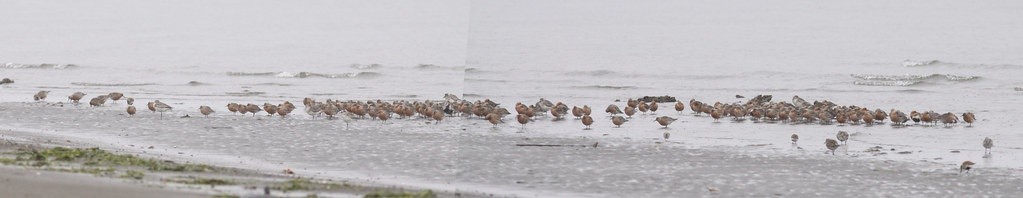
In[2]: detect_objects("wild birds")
[89,92,136,118]
[33,90,52,102]
[605,97,658,128]
[982,136,993,154]
[824,130,849,156]
[652,115,678,128]
[0,77,15,85]
[960,160,976,174]
[690,94,977,128]
[675,100,684,114]
[791,133,799,144]
[198,105,214,118]
[225,101,296,118]
[303,93,511,127]
[146,100,172,120]
[68,91,87,102]
[515,98,595,130]
